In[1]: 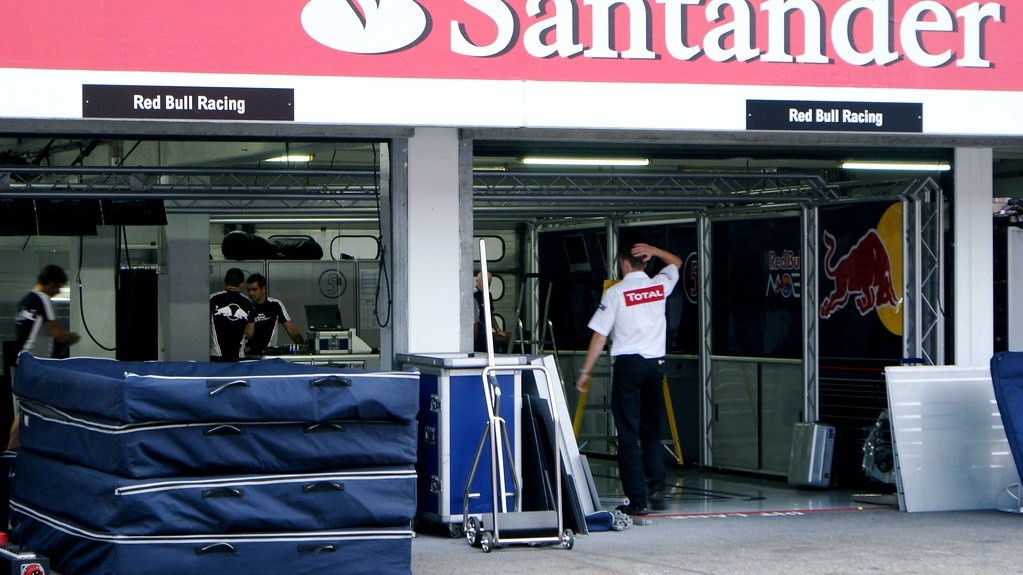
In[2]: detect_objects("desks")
[244,354,380,371]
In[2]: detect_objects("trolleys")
[460,366,574,554]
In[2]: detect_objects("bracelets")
[578,370,592,375]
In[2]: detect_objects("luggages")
[788,421,836,489]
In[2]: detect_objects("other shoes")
[615,505,649,514]
[647,496,672,510]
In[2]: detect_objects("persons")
[210,268,305,362]
[474,271,506,352]
[575,241,682,515]
[6,264,81,452]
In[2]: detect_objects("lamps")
[513,156,652,167]
